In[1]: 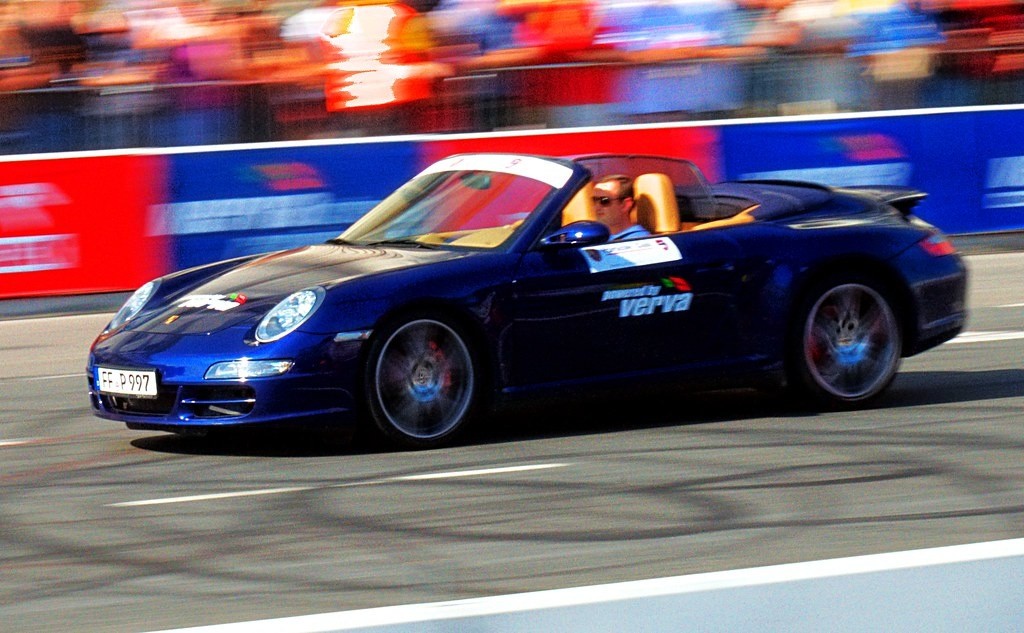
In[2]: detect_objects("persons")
[591,174,651,242]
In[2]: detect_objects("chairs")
[627,171,682,237]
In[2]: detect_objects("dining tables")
[559,176,601,235]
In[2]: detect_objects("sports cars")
[85,146,970,451]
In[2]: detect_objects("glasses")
[592,195,623,206]
